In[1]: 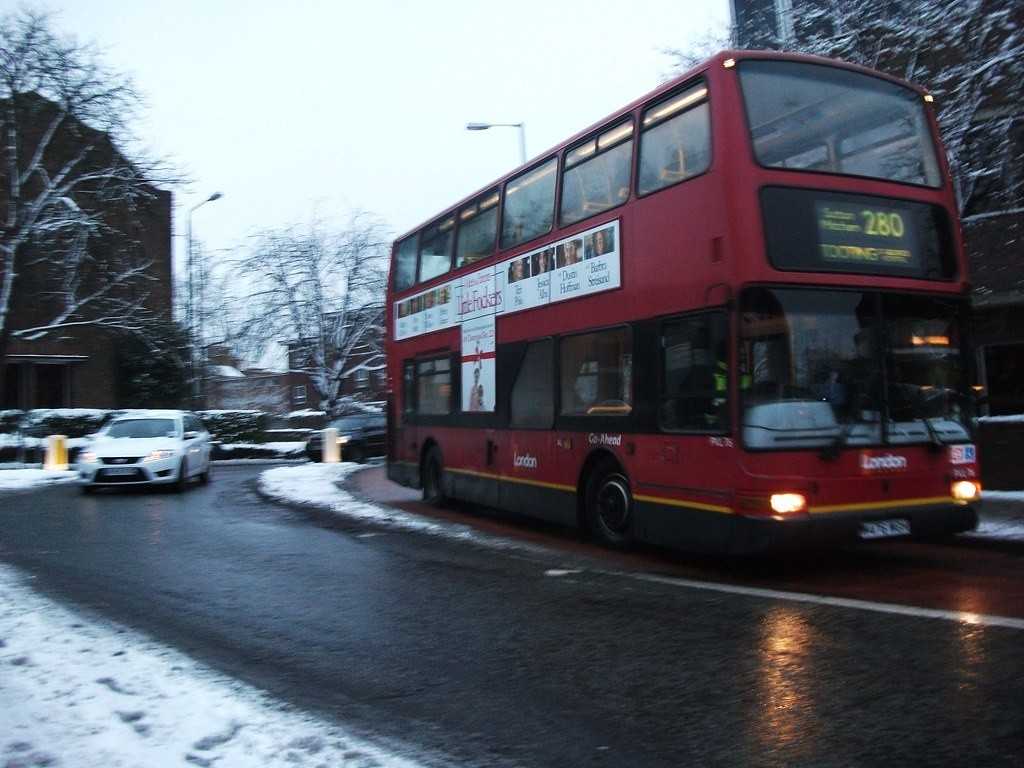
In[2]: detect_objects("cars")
[79,413,217,494]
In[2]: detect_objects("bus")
[383,49,984,551]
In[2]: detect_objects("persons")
[397,232,612,318]
[698,344,750,429]
[468,369,487,410]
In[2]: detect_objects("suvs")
[305,410,386,463]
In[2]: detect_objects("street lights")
[464,122,526,165]
[188,194,217,404]
